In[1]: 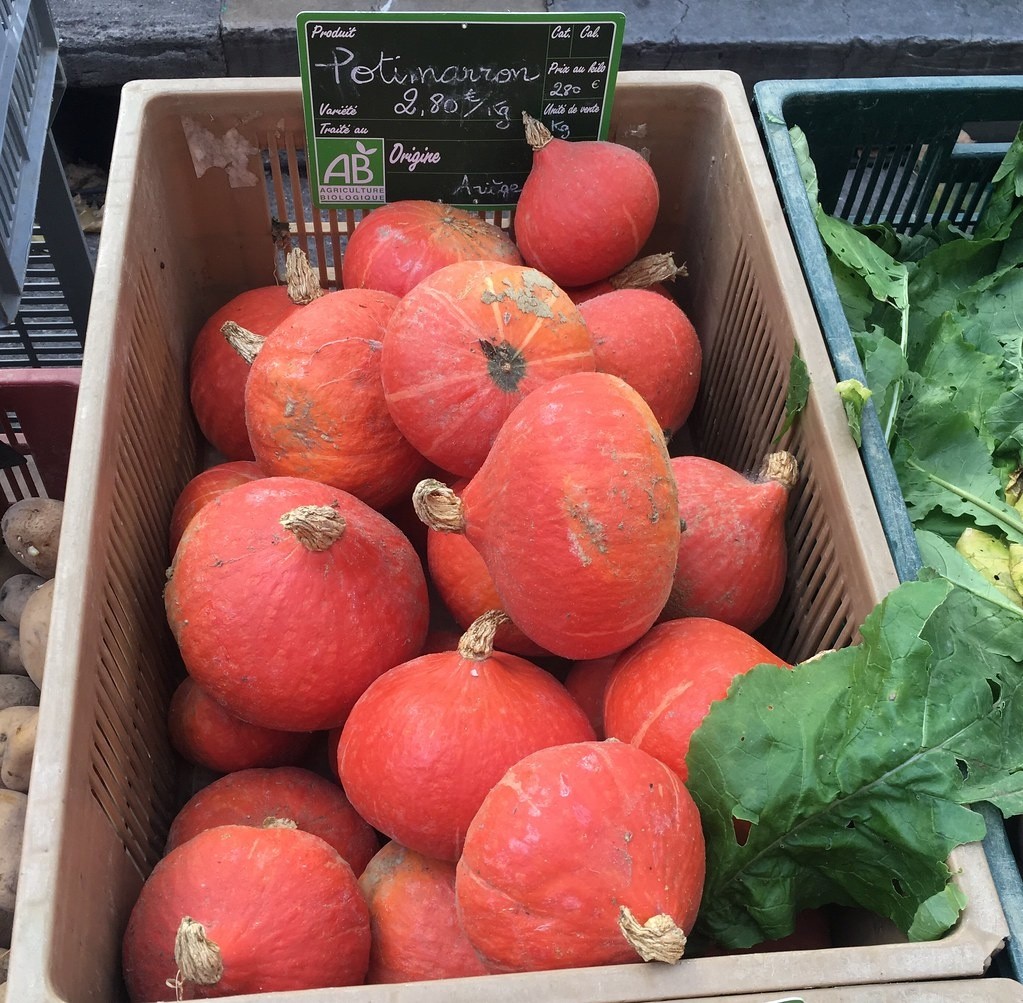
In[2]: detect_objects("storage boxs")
[0,0,1023,1003]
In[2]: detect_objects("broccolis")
[683,124,1023,952]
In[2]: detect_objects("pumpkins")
[121,110,795,1003]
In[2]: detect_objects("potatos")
[0,495,63,988]
